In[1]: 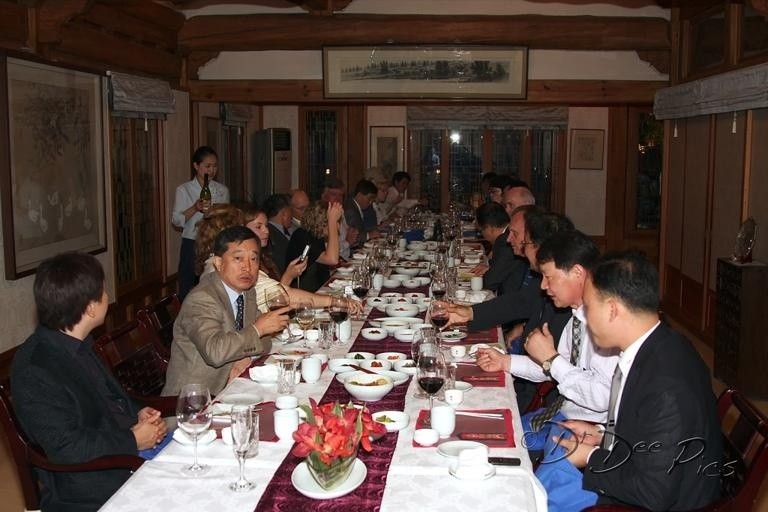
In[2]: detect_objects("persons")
[9,251,174,512]
[161,146,724,511]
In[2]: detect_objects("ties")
[528,315,585,434]
[602,364,624,450]
[233,296,245,333]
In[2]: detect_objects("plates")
[263,355,303,366]
[385,304,419,317]
[291,457,367,500]
[436,440,490,458]
[334,370,408,384]
[377,303,427,313]
[279,348,312,355]
[436,331,467,342]
[206,403,254,420]
[220,393,262,405]
[367,317,424,328]
[340,404,370,413]
[294,307,339,320]
[455,380,472,392]
[450,462,495,480]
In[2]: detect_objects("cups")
[335,317,352,342]
[274,410,299,439]
[277,359,297,394]
[301,358,322,383]
[471,276,483,290]
[373,274,383,288]
[437,365,456,401]
[234,412,261,459]
[317,322,334,350]
[430,406,455,438]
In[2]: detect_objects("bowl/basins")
[390,298,412,304]
[416,298,431,306]
[380,321,410,336]
[404,293,425,302]
[344,375,393,401]
[328,359,358,372]
[381,293,404,301]
[394,329,417,343]
[222,426,234,445]
[389,274,413,282]
[287,370,301,384]
[402,280,421,288]
[305,330,318,342]
[359,359,392,371]
[175,429,216,446]
[353,253,365,260]
[328,283,343,292]
[376,352,407,368]
[275,396,298,410]
[367,297,389,307]
[344,352,375,359]
[337,267,353,277]
[446,390,464,405]
[362,328,387,341]
[455,290,465,300]
[412,277,431,286]
[451,346,465,359]
[393,238,436,275]
[334,279,351,285]
[413,428,440,446]
[410,324,433,329]
[384,280,401,289]
[309,354,328,366]
[249,365,279,385]
[394,359,420,375]
[372,411,410,432]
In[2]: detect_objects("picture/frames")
[1,48,107,279]
[321,45,527,101]
[570,129,603,168]
[369,125,405,186]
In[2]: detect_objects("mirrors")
[731,217,758,263]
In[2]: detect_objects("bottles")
[200,174,212,213]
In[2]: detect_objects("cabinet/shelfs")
[714,259,766,398]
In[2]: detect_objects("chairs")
[580,388,766,509]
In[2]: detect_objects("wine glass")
[295,296,314,348]
[351,272,371,321]
[176,384,212,475]
[429,205,460,294]
[417,350,448,425]
[396,206,425,228]
[228,405,257,492]
[327,294,349,346]
[411,328,442,398]
[430,296,449,351]
[264,282,303,345]
[361,226,404,272]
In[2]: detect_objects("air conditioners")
[249,126,291,207]
